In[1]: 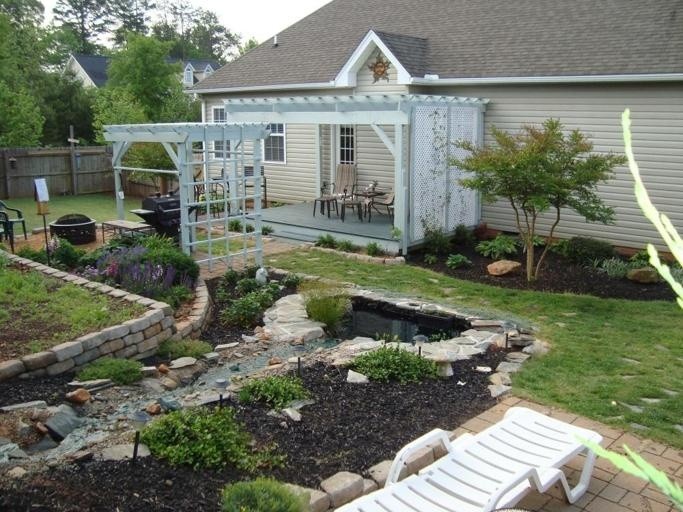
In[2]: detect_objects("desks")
[101,220,152,245]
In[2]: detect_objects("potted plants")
[447,224,474,256]
[391,228,401,239]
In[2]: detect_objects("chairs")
[0,200,27,255]
[312,164,395,227]
[331,404,603,512]
